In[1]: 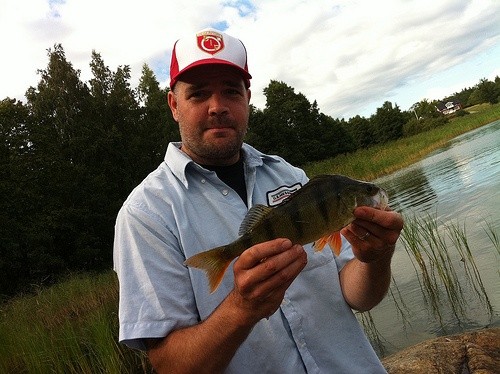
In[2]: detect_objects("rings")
[360,231,371,240]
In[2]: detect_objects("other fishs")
[181,174,389,295]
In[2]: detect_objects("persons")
[112,26,405,374]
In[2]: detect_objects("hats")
[170,29,252,92]
